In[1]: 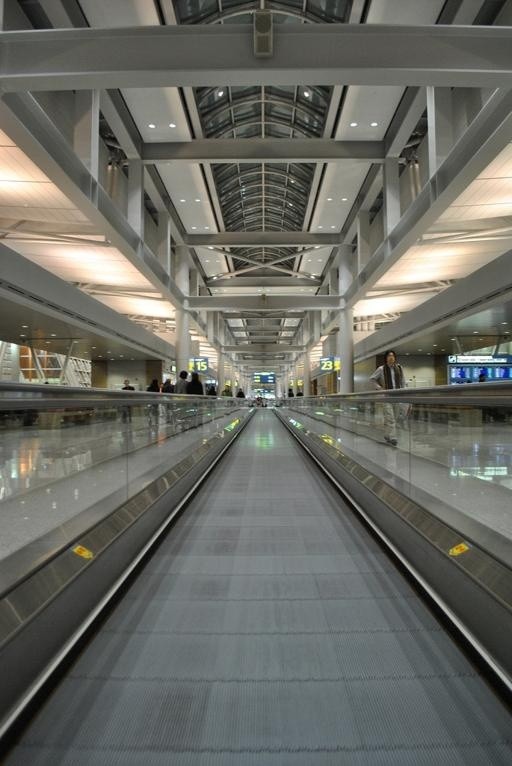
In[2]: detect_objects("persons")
[186,372,204,396]
[161,378,175,395]
[479,373,486,382]
[145,378,160,430]
[173,370,189,395]
[287,387,295,398]
[236,388,245,398]
[208,386,217,396]
[221,384,233,397]
[118,379,136,425]
[367,349,406,448]
